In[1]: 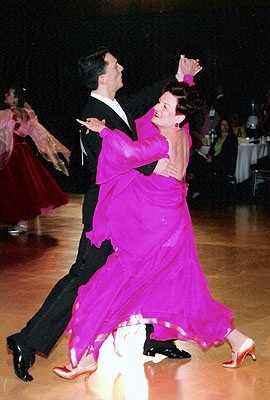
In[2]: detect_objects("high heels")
[222,337,256,368]
[54,360,97,381]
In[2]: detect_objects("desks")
[188,134,270,184]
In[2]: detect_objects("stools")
[212,175,237,208]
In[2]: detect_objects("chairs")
[251,169,270,206]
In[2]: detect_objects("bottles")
[237,128,242,137]
[210,129,215,145]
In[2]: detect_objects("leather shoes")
[143,341,192,359]
[7,334,33,381]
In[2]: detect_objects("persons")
[6,45,202,382]
[0,86,71,228]
[187,85,270,209]
[53,54,257,381]
